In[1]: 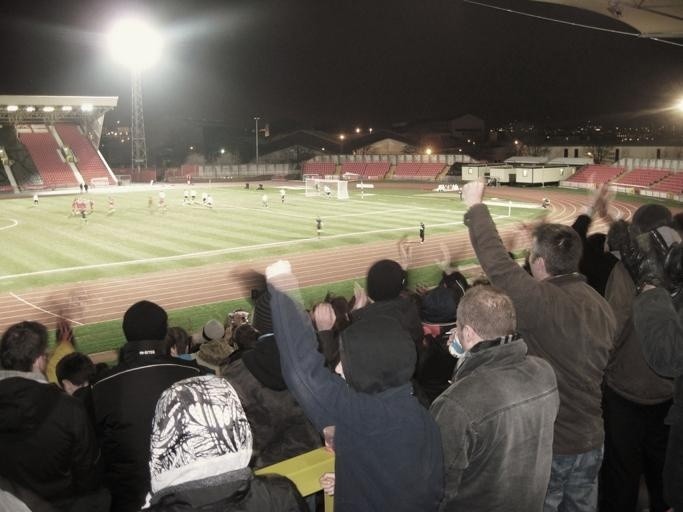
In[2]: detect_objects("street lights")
[339,134,345,154]
[103,13,161,168]
[253,117,261,176]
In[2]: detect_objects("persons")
[0,180,682,510]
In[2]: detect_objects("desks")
[567,163,683,200]
[302,157,448,180]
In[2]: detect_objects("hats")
[123,302,168,342]
[367,261,467,326]
[197,291,298,369]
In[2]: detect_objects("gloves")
[621,229,683,293]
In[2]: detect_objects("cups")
[448,332,464,359]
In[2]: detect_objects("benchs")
[15,121,116,191]
